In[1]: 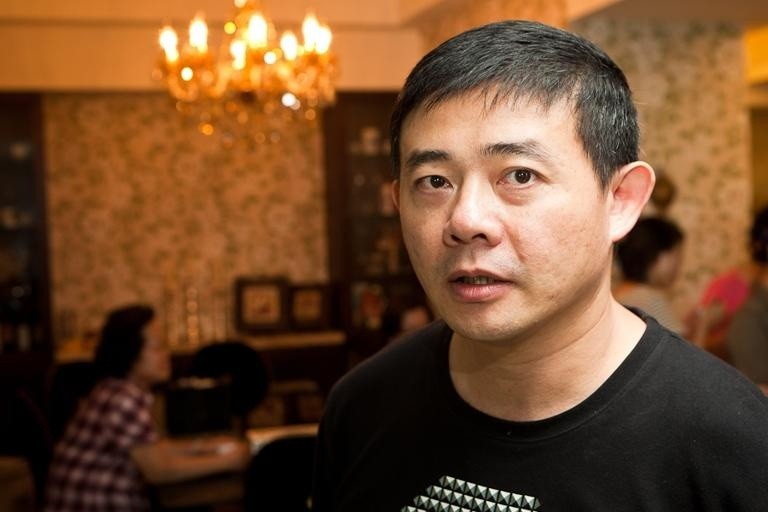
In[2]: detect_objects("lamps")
[150,0,337,137]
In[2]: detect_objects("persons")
[725,236,767,397]
[44,305,251,510]
[686,203,767,367]
[610,217,693,343]
[302,21,768,512]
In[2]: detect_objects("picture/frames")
[284,280,329,331]
[231,273,285,336]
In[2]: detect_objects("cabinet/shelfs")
[319,85,439,368]
[0,90,57,462]
